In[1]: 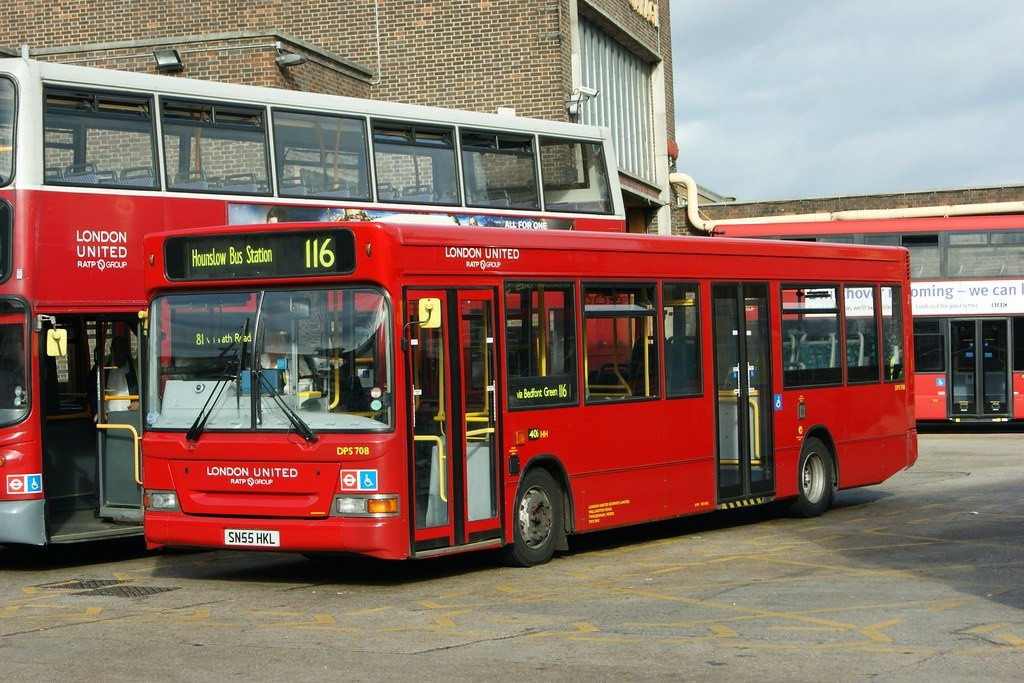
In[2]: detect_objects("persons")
[87,336,138,519]
[257,330,313,394]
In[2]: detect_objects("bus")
[1,55,626,548]
[135,222,919,566]
[711,215,1024,427]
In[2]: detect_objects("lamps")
[152,48,183,72]
[276,41,308,67]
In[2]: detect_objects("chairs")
[599,332,697,395]
[782,325,899,370]
[46,162,582,212]
